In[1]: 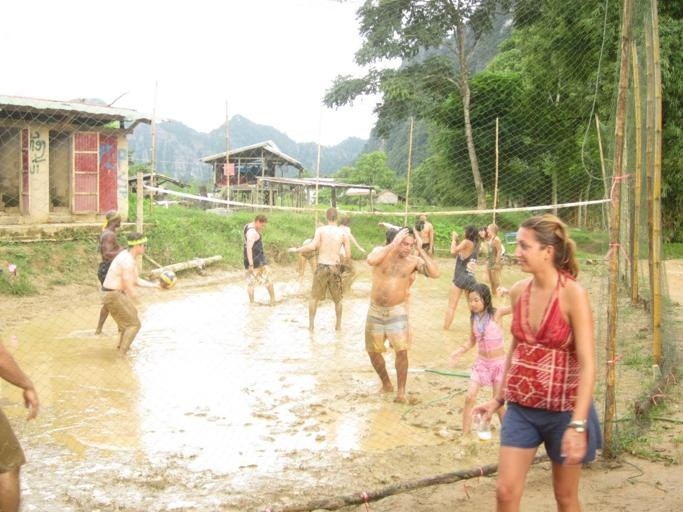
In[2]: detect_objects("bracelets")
[567,420,588,432]
[459,345,467,352]
[494,396,505,405]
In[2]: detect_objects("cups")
[474,414,493,441]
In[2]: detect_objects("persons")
[242,215,275,305]
[442,223,481,331]
[296,214,506,296]
[0,340,40,510]
[99,231,162,358]
[366,225,442,403]
[287,207,351,332]
[473,213,604,512]
[93,209,125,337]
[451,282,514,434]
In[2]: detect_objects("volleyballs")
[160,271,177,287]
